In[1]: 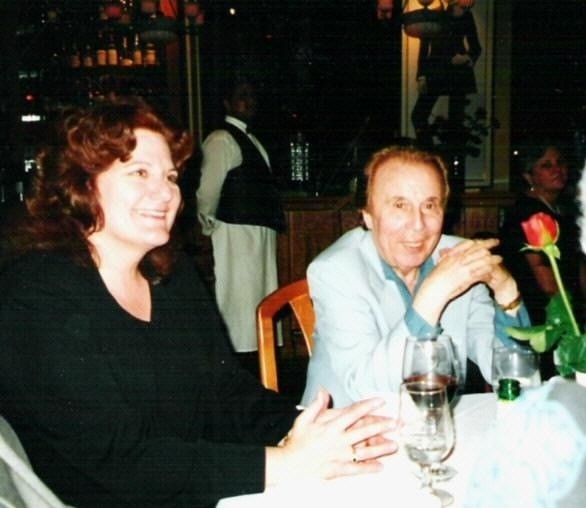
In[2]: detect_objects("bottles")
[71,29,160,103]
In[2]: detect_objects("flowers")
[505,212,586,378]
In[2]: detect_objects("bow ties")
[246,126,257,134]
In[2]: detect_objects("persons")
[411,8,482,145]
[196,80,284,376]
[301,145,535,420]
[2,94,403,508]
[499,140,585,380]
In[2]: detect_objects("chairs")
[0,417,72,508]
[256,279,316,392]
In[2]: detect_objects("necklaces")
[537,192,561,214]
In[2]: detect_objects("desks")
[214,382,586,508]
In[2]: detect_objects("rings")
[353,449,356,462]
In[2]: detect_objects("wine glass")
[397,331,459,508]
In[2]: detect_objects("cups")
[489,345,541,408]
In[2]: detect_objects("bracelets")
[502,294,522,311]
